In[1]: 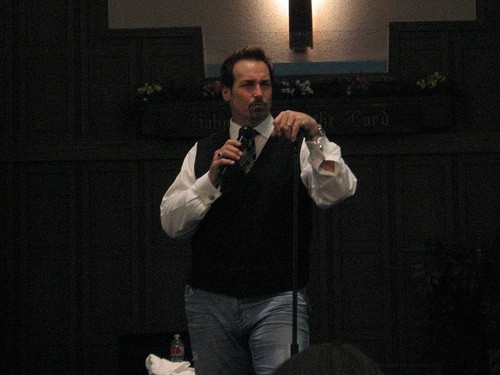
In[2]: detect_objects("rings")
[218,151,223,159]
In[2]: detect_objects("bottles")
[170,334,184,362]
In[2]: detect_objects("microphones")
[221,125,254,177]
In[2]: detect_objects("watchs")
[305,125,325,141]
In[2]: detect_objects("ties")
[237,128,260,174]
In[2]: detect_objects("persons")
[271,343,386,375]
[159,46,357,375]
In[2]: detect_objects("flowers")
[343,72,369,96]
[202,80,222,100]
[137,81,163,103]
[415,72,449,91]
[279,79,314,97]
